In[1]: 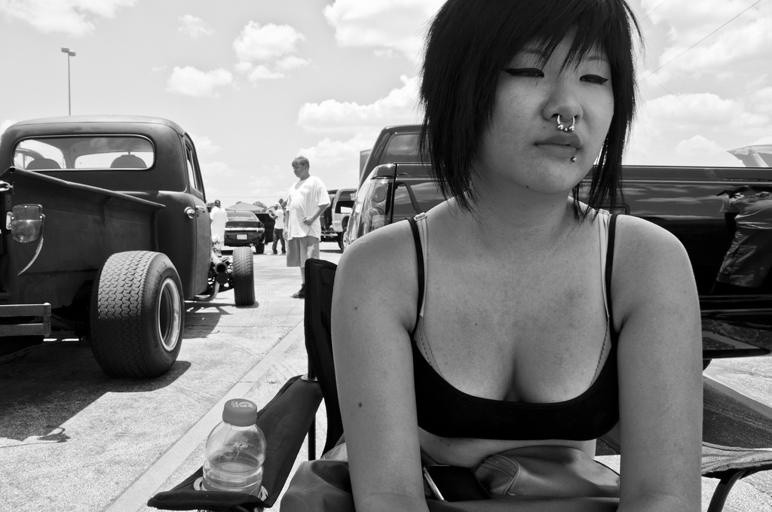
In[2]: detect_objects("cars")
[321,187,358,249]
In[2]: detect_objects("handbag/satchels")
[281,442,621,512]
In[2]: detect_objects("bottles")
[199,398,266,494]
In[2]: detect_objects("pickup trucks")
[342,119,771,371]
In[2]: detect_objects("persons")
[279,199,289,240]
[286,156,333,299]
[268,203,287,254]
[211,199,229,247]
[282,0,703,512]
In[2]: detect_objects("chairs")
[147,256,770,511]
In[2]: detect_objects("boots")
[292,284,306,298]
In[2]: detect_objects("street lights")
[61,44,75,115]
